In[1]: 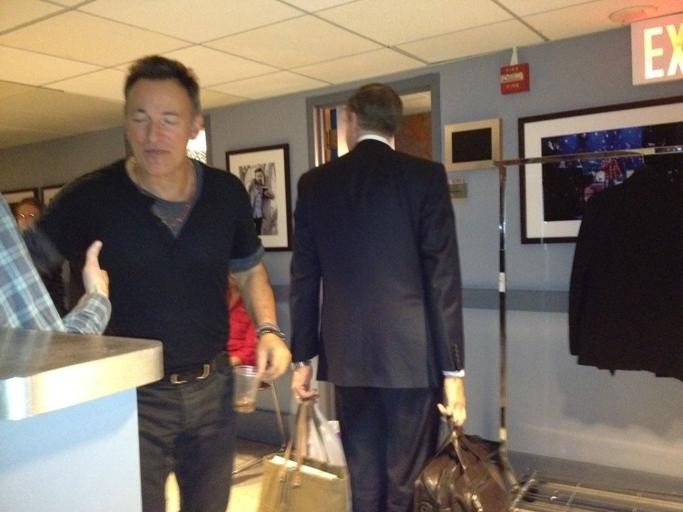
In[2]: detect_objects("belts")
[153,354,228,386]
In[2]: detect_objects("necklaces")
[131,156,196,229]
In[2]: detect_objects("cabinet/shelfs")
[1,329,166,511]
[2,184,73,212]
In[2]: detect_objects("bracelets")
[291,358,311,370]
[253,320,287,340]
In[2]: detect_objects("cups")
[232,365,262,413]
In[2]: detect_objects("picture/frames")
[225,146,289,252]
[517,96,682,247]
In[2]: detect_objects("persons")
[246,167,276,234]
[24,50,293,512]
[224,272,268,389]
[0,192,113,337]
[289,82,470,509]
[12,197,68,317]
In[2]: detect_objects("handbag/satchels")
[409,415,517,512]
[255,397,354,512]
